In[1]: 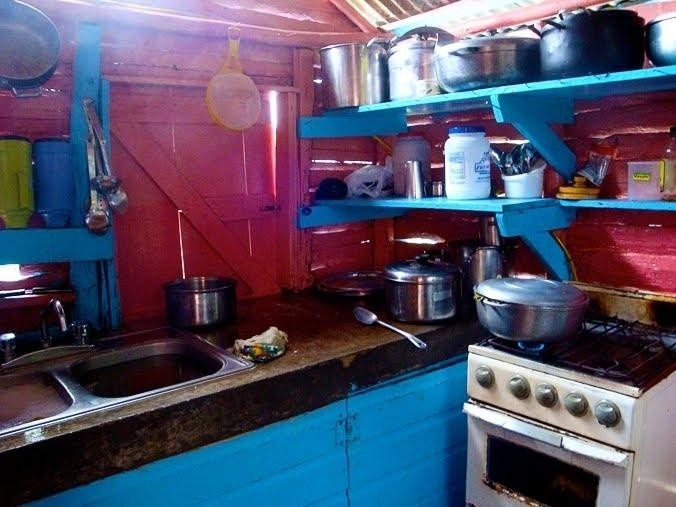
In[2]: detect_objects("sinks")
[66,334,229,402]
[0,366,75,435]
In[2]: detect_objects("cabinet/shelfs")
[294,64,676,283]
[0,20,123,346]
[0,353,676,507]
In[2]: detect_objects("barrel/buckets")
[320,42,366,110]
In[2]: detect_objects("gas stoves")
[467,316,676,398]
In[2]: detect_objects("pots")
[433,31,543,97]
[534,10,645,80]
[387,33,438,99]
[0,1,62,99]
[647,11,676,69]
[379,239,589,343]
[162,275,238,331]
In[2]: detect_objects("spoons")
[84,96,130,214]
[354,306,427,350]
[490,142,537,175]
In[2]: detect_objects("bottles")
[661,127,676,201]
[392,126,490,199]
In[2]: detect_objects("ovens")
[462,352,635,507]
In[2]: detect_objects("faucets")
[38,297,71,349]
